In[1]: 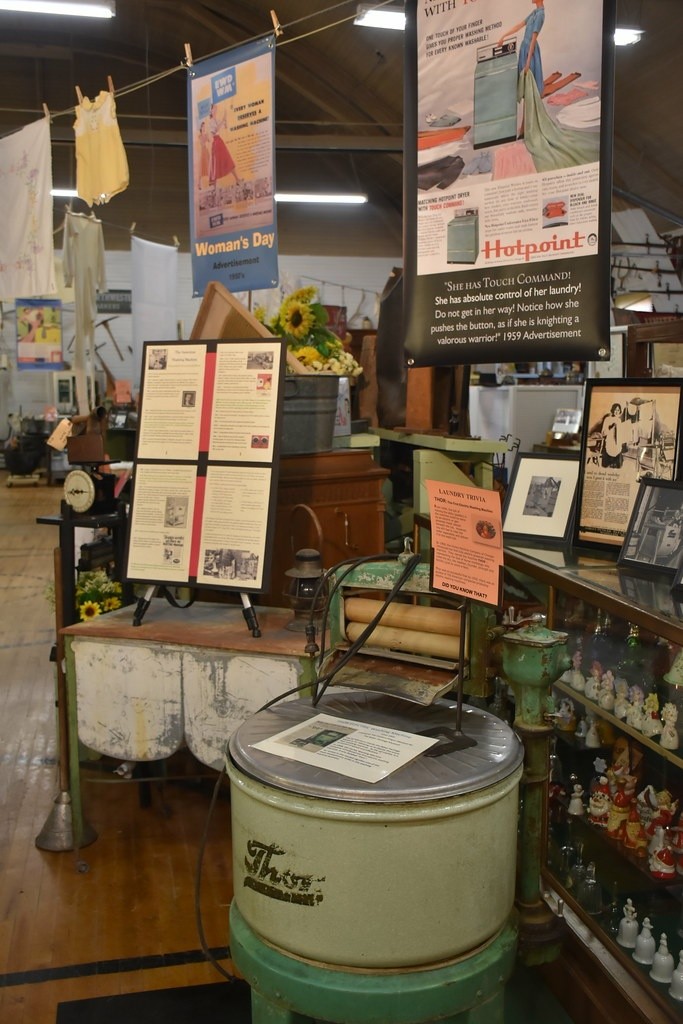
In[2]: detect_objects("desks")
[59,596,332,850]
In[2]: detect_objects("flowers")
[70,566,128,620]
[246,281,365,384]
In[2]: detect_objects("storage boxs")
[468,382,589,453]
[332,376,351,436]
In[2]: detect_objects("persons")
[497,0,545,140]
[601,404,628,468]
[196,102,245,192]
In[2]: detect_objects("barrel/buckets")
[224,738,524,974]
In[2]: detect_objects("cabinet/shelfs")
[184,446,391,621]
[501,540,683,1024]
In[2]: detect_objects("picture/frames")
[618,475,683,577]
[573,378,683,553]
[502,452,582,552]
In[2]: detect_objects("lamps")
[0,0,117,19]
[353,3,646,47]
